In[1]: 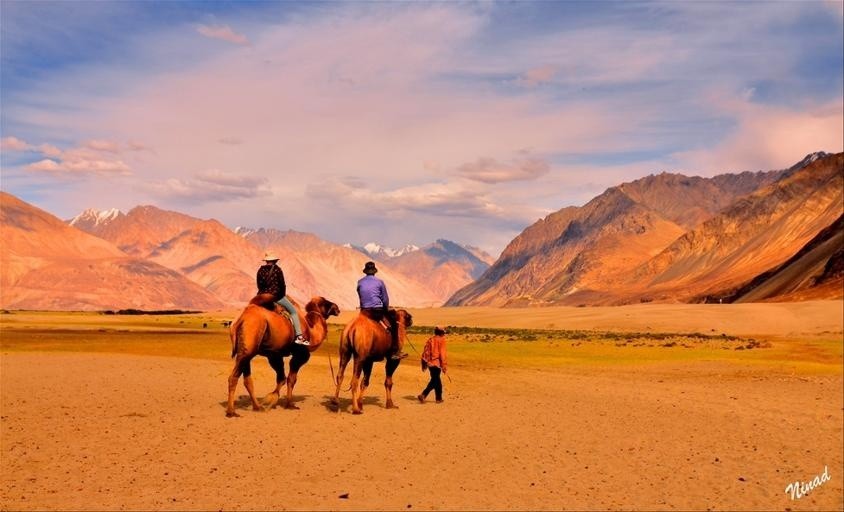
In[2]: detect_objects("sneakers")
[294,335,310,345]
[418,393,444,404]
[391,353,409,360]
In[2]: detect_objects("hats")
[363,262,377,274]
[261,252,280,261]
[434,325,445,331]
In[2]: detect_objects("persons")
[418,325,447,403]
[356,261,408,360]
[256,251,310,346]
